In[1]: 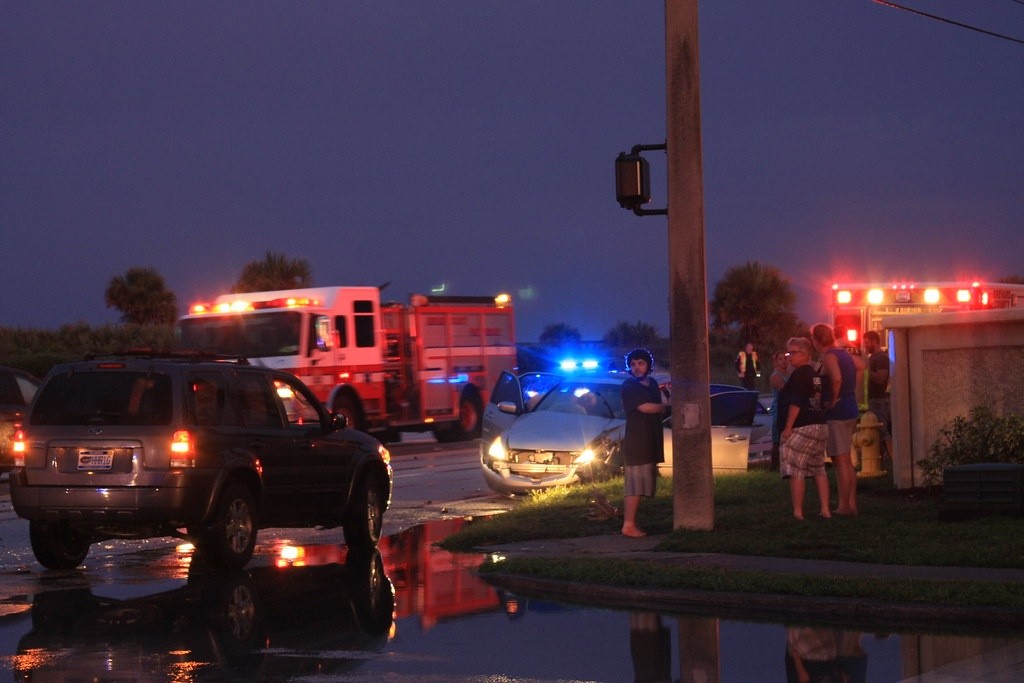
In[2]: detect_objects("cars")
[478,356,774,499]
[0,366,54,473]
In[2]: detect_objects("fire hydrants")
[852,409,889,478]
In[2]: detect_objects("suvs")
[7,346,392,570]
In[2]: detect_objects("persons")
[769,323,894,520]
[280,321,299,354]
[734,343,762,388]
[621,348,673,537]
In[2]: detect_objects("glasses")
[785,351,804,358]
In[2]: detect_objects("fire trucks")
[180,284,518,445]
[829,281,1020,349]
